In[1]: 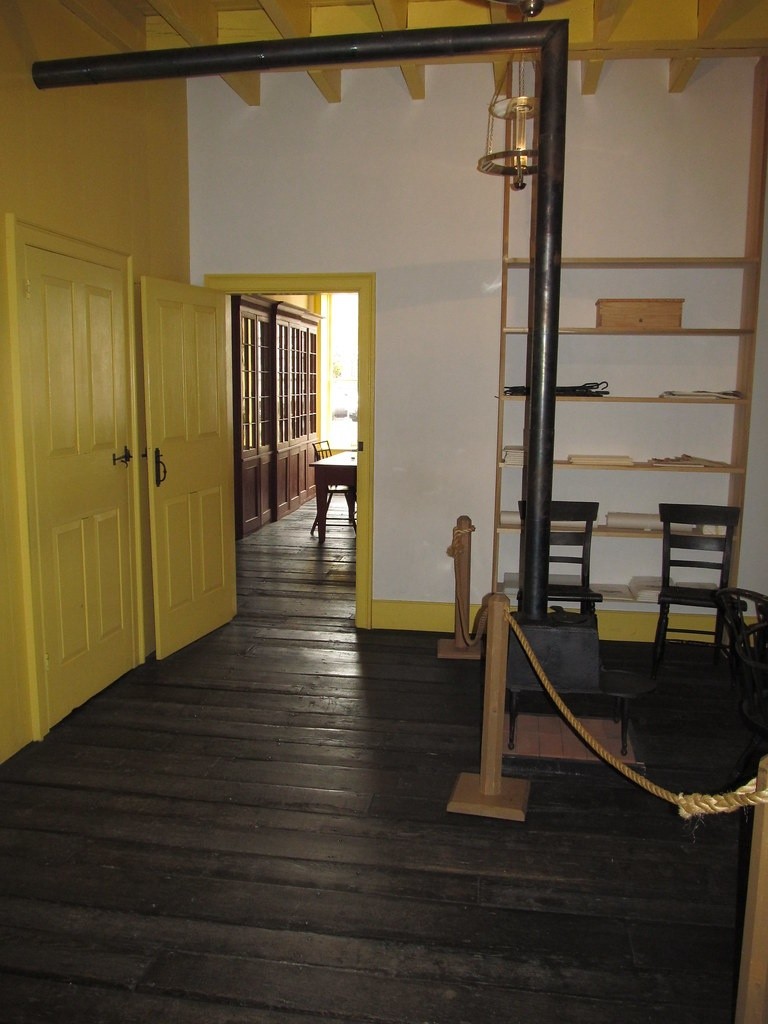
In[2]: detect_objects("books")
[503,572,719,607]
[567,454,635,467]
[500,511,521,525]
[658,390,744,400]
[702,525,727,536]
[648,454,737,469]
[605,510,667,530]
[502,445,524,465]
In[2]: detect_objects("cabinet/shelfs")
[229,294,279,538]
[301,310,324,501]
[490,256,762,601]
[273,301,306,521]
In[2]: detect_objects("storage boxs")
[594,300,684,327]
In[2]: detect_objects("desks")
[309,451,358,544]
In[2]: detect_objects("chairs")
[309,440,357,535]
[715,588,768,793]
[515,499,603,613]
[506,619,659,755]
[651,503,741,689]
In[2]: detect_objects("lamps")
[477,15,540,190]
